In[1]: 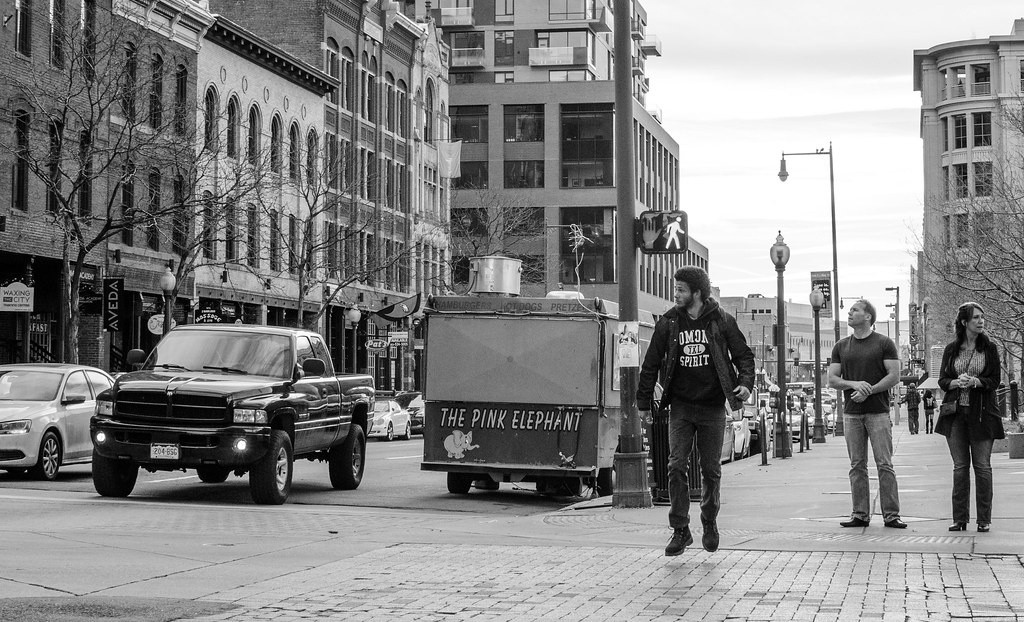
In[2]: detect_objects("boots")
[930,418,933,434]
[925,420,929,434]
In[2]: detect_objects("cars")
[366,391,425,440]
[721,381,844,462]
[0,362,118,480]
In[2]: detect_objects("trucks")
[420,294,662,500]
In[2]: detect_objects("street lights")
[348,303,362,374]
[778,141,845,436]
[809,285,827,443]
[159,266,175,336]
[885,287,900,425]
[769,230,792,457]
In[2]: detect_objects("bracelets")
[972,377,976,388]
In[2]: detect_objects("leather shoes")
[884,519,907,529]
[839,515,868,528]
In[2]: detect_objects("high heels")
[977,519,990,532]
[949,518,967,531]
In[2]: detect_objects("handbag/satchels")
[938,401,957,414]
[932,400,938,408]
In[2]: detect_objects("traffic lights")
[641,210,688,253]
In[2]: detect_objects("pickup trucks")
[90,322,376,506]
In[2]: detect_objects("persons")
[905,383,921,434]
[636,265,756,557]
[829,299,908,528]
[934,302,1005,531]
[923,390,939,434]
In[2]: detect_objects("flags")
[370,293,421,330]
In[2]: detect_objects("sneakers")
[699,513,718,551]
[664,524,694,555]
